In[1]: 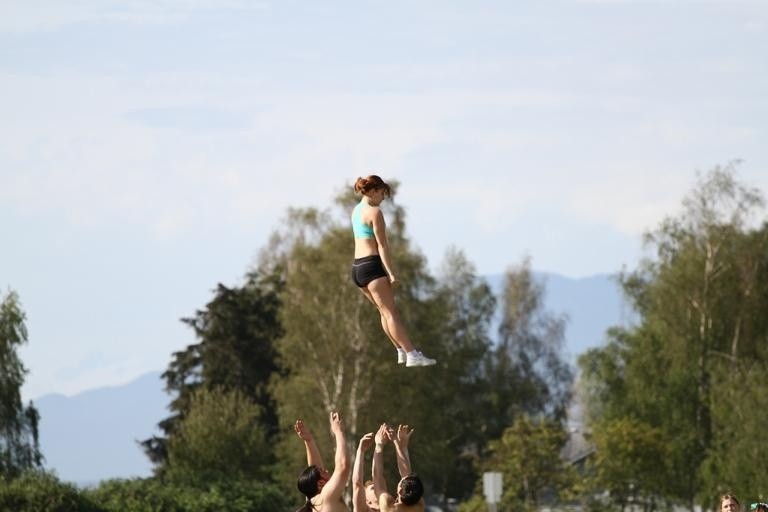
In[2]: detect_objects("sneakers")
[397,350,437,367]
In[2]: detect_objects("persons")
[352,424,414,511]
[351,175,438,368]
[293,411,350,511]
[718,494,744,511]
[750,503,768,512]
[370,423,427,512]
